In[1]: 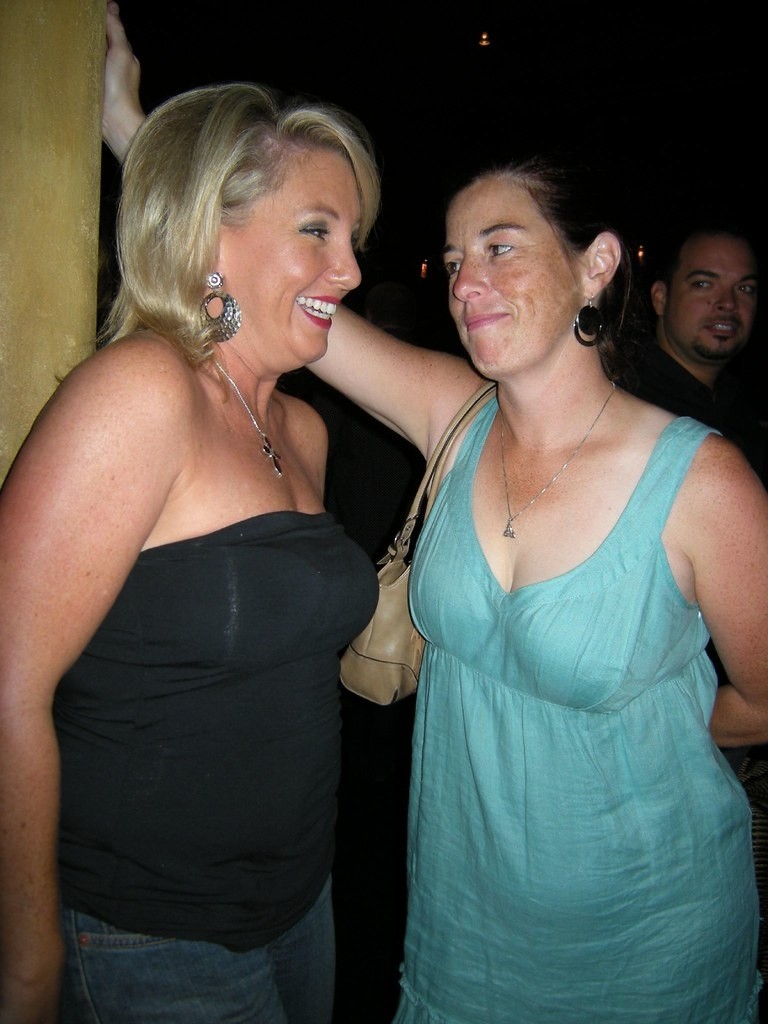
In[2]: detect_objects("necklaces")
[499,381,617,538]
[211,355,285,477]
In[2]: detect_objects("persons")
[2,81,381,1022]
[601,217,767,776]
[102,1,768,1023]
[272,279,476,563]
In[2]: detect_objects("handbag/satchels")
[339,380,497,707]
